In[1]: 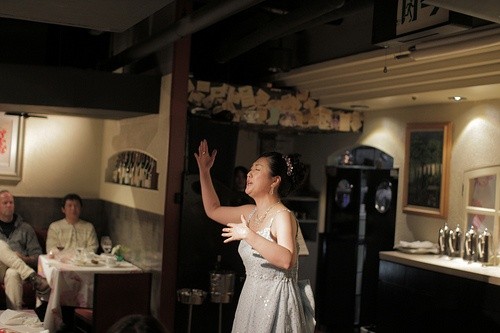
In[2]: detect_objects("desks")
[0,309,50,333]
[36,254,143,333]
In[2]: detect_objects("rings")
[229,229,230,233]
[203,152,205,153]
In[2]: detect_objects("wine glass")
[56,238,65,253]
[101,236,113,253]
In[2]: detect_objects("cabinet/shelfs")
[374,263,500,333]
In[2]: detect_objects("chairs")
[73,272,152,333]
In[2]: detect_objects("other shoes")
[28,273,51,295]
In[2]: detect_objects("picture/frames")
[0,112,25,181]
[402,123,453,219]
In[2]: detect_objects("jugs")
[439,222,492,262]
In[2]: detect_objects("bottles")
[112,151,157,190]
[212,254,224,274]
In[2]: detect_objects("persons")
[46,193,99,254]
[0,190,52,310]
[194,139,317,333]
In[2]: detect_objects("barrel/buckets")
[208,269,236,303]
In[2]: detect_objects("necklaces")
[256,200,280,225]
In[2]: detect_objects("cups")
[76,248,84,255]
[107,256,117,265]
